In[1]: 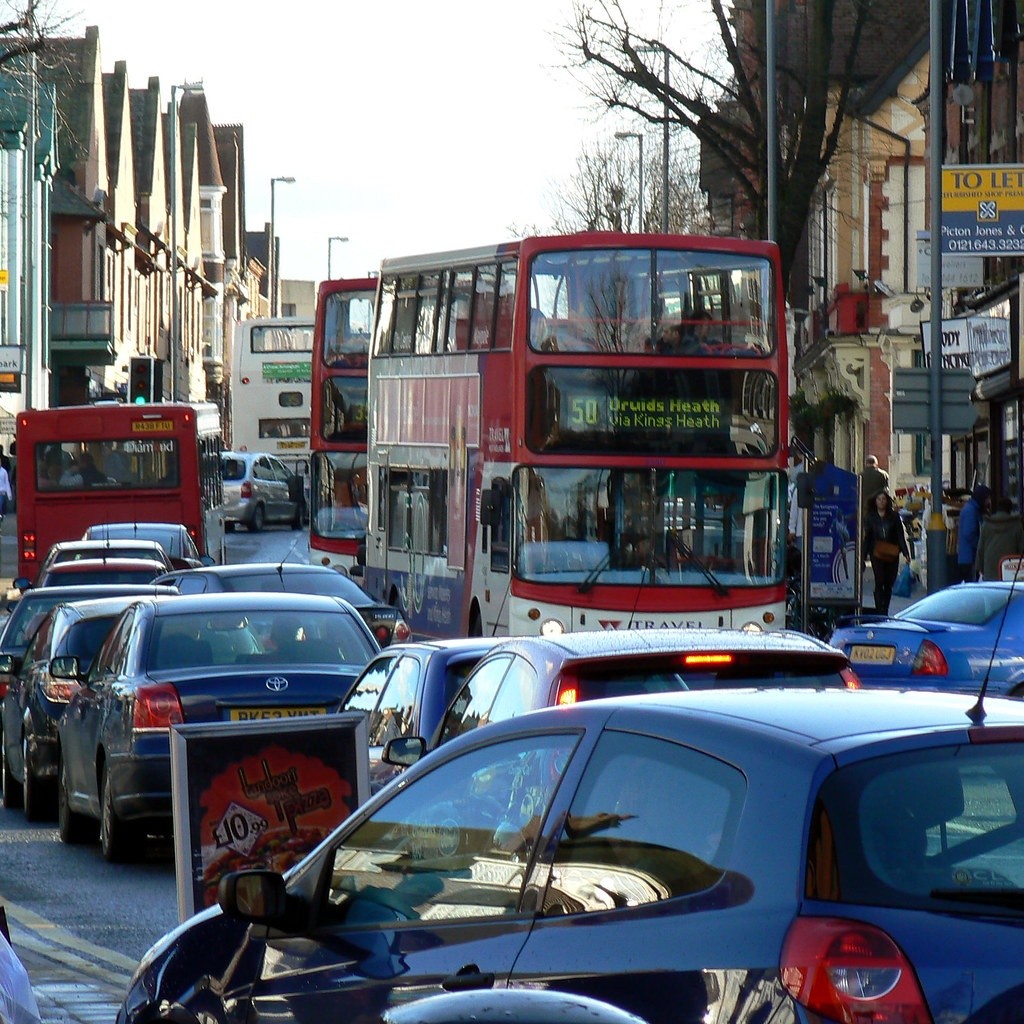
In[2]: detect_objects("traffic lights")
[128,356,155,406]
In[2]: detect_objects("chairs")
[875,761,966,852]
[284,637,343,664]
[537,342,761,356]
[157,640,212,672]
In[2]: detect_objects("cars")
[0,523,1024,1023]
[219,449,305,534]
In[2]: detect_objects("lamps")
[851,267,869,281]
[909,282,931,314]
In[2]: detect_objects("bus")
[16,404,238,592]
[307,230,821,643]
[230,316,318,494]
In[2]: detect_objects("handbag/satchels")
[891,562,913,598]
[873,540,900,563]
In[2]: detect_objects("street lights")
[169,83,204,403]
[632,44,670,233]
[270,177,296,316]
[328,237,349,281]
[614,131,644,235]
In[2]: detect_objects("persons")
[859,454,910,616]
[656,310,712,356]
[0,434,131,514]
[957,485,1024,585]
